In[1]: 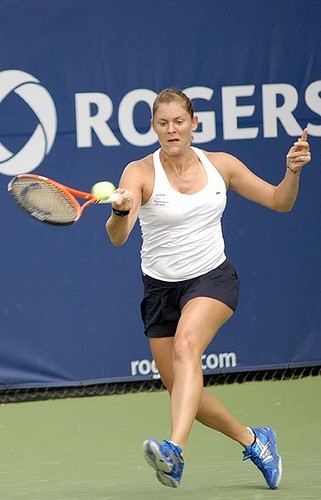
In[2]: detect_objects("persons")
[105,88,313,489]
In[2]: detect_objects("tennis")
[92,181,115,201]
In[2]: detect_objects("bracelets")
[112,208,129,217]
[287,166,302,175]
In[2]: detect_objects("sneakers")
[142,438,185,488]
[242,425,282,490]
